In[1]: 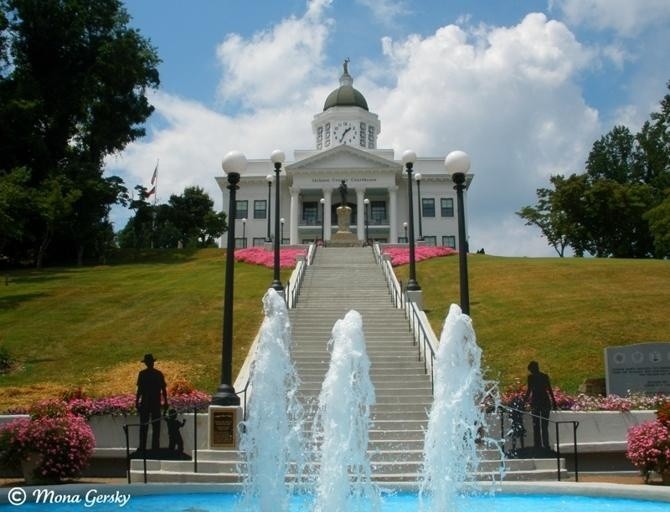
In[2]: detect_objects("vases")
[20,453,45,484]
[660,465,669,486]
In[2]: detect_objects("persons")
[338,180,348,207]
[163,406,186,452]
[523,360,558,451]
[505,395,527,449]
[129,353,169,456]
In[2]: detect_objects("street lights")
[264,173,274,250]
[444,150,471,316]
[207,150,248,405]
[241,218,248,249]
[403,222,409,246]
[401,149,425,291]
[279,216,287,246]
[362,199,372,247]
[319,197,328,246]
[269,147,285,290]
[414,173,427,248]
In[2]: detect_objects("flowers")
[623,417,670,482]
[1,379,212,417]
[0,397,97,479]
[478,379,669,427]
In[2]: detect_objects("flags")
[150,165,158,184]
[144,187,156,198]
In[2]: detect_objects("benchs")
[543,409,658,472]
[84,413,207,480]
